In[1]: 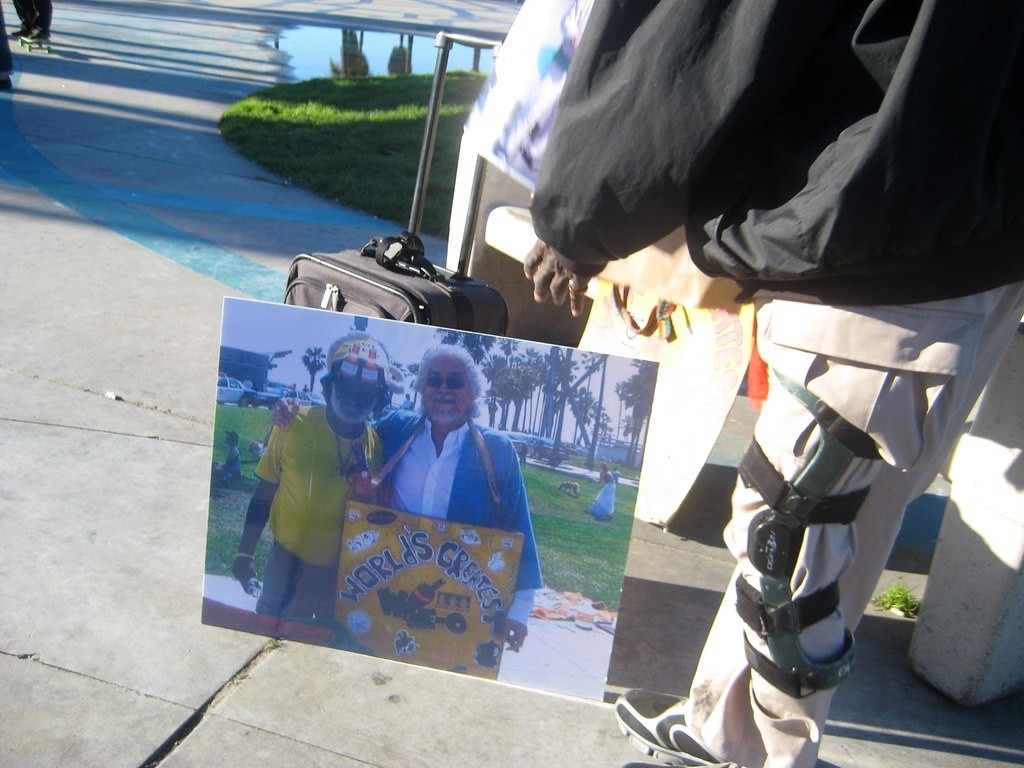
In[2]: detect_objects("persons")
[525,1,1023,768]
[0,0,14,89]
[231,332,544,653]
[11,0,53,38]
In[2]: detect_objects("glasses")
[424,377,466,390]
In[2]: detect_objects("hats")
[320,338,390,390]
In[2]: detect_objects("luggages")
[284,233,508,336]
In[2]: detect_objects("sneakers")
[616,687,726,767]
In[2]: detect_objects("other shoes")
[12,26,50,39]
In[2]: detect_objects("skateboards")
[18,33,52,54]
